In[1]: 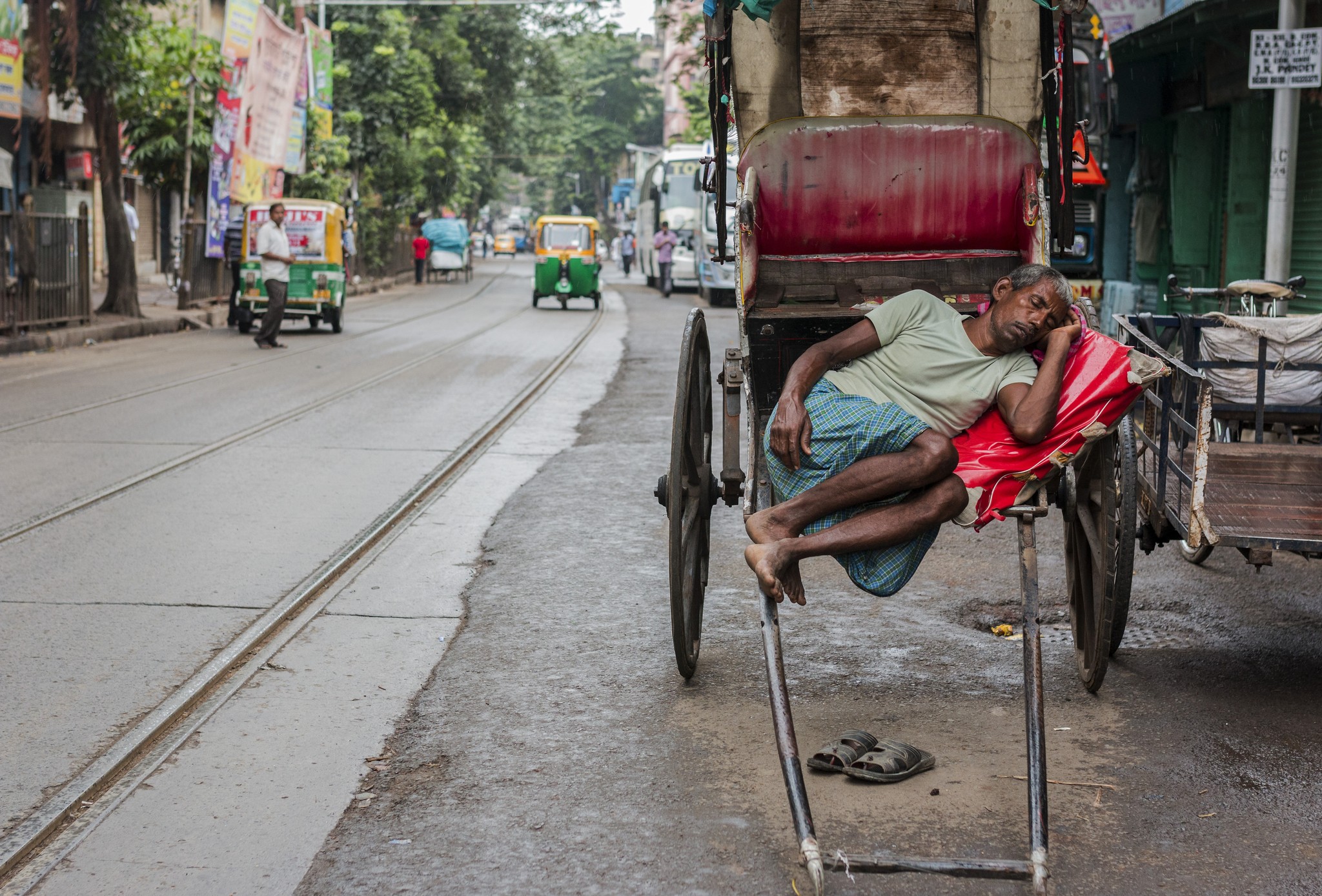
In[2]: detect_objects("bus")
[633,141,722,290]
[690,140,740,303]
[1050,1,1119,311]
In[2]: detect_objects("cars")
[494,236,515,256]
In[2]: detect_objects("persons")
[482,239,487,256]
[742,263,1082,607]
[653,222,678,298]
[223,203,252,327]
[254,203,296,348]
[123,192,140,242]
[621,232,634,275]
[412,230,430,285]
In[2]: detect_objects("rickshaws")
[651,0,1154,896]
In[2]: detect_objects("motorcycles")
[531,215,606,310]
[239,198,351,334]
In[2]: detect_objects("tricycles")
[1112,273,1320,658]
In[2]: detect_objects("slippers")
[270,341,288,348]
[254,337,273,350]
[806,728,935,784]
